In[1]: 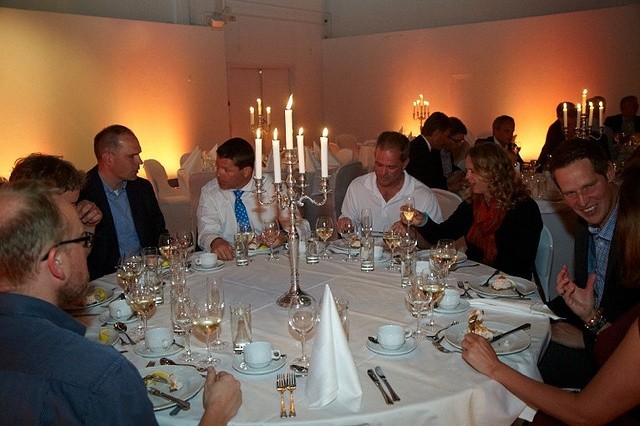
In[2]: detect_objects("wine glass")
[262,218,281,262]
[382,224,402,271]
[116,248,147,307]
[287,293,318,376]
[157,229,193,287]
[314,215,334,260]
[400,196,416,243]
[340,217,360,263]
[190,299,224,369]
[420,272,445,327]
[404,275,431,339]
[129,279,158,341]
[437,238,459,276]
[237,219,256,244]
[360,208,373,243]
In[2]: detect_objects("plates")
[282,250,308,258]
[84,325,121,347]
[61,284,115,310]
[134,336,186,358]
[374,250,392,263]
[137,364,204,411]
[191,260,226,272]
[366,336,417,356]
[433,299,471,314]
[416,249,467,264]
[443,320,531,356]
[231,352,287,375]
[331,239,376,252]
[99,309,139,325]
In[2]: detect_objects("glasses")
[451,136,466,147]
[41,230,96,264]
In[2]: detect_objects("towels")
[187,149,202,171]
[467,297,563,321]
[182,146,199,169]
[307,283,364,413]
[208,143,217,161]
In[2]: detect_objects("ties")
[233,190,251,234]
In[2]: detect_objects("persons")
[474,115,525,166]
[399,141,544,281]
[459,147,640,426]
[604,95,639,134]
[536,100,613,163]
[404,111,474,245]
[7,153,103,259]
[333,131,444,250]
[581,95,613,156]
[547,136,640,350]
[196,135,311,262]
[0,178,243,425]
[76,124,175,281]
[434,116,467,182]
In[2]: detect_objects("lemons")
[99,329,109,342]
[95,288,106,301]
[150,371,169,378]
[162,260,169,267]
[258,245,268,249]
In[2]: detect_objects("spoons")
[425,327,451,341]
[367,331,409,344]
[160,358,209,373]
[432,342,462,354]
[113,321,137,346]
[171,338,186,349]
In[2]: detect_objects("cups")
[377,324,413,351]
[169,285,194,335]
[234,233,249,266]
[242,342,281,369]
[230,302,253,354]
[141,246,165,307]
[305,229,321,264]
[108,299,136,321]
[514,158,550,199]
[428,244,450,279]
[360,238,374,271]
[144,327,174,353]
[415,261,431,277]
[206,275,226,318]
[284,241,306,256]
[374,246,384,260]
[334,299,350,343]
[437,289,461,310]
[195,253,218,268]
[401,248,420,288]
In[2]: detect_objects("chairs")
[143,158,189,232]
[432,188,464,252]
[306,160,363,238]
[180,152,191,167]
[531,224,553,302]
[336,148,353,163]
[335,134,360,151]
[191,171,219,249]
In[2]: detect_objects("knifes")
[375,366,401,401]
[367,368,395,405]
[147,387,190,410]
[488,322,531,343]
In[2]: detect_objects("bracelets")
[584,307,609,334]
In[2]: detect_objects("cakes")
[492,276,513,291]
[467,310,502,341]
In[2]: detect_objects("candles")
[416,99,420,114]
[253,127,264,193]
[575,102,581,133]
[424,100,427,114]
[271,127,283,192]
[413,102,416,115]
[599,101,604,130]
[319,127,331,187]
[587,101,594,130]
[427,101,430,114]
[419,93,424,107]
[296,127,308,187]
[266,106,271,124]
[283,93,296,164]
[581,88,587,118]
[249,105,254,124]
[257,97,262,114]
[562,102,568,131]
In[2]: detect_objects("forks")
[286,372,297,418]
[457,281,473,299]
[276,373,286,419]
[479,269,500,287]
[437,321,460,343]
